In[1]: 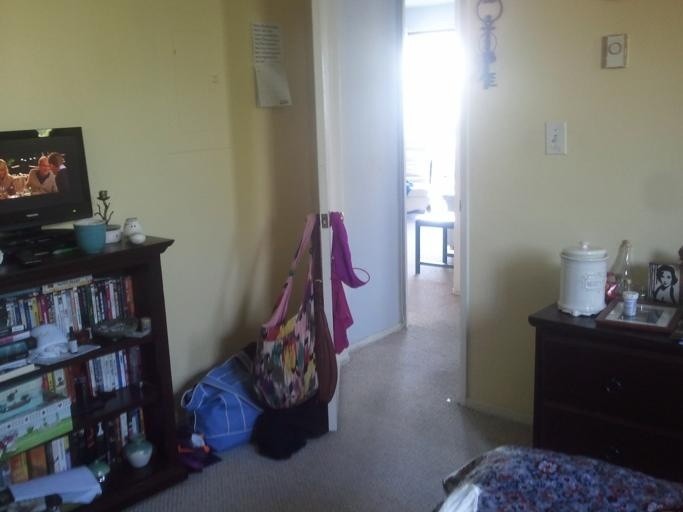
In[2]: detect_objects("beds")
[432,441,683,512]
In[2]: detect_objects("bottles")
[621,239,632,293]
[74,375,89,410]
[67,326,77,354]
[88,459,112,497]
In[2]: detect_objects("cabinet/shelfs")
[0,226,192,510]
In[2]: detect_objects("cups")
[622,290,638,317]
[140,319,150,332]
[72,219,106,254]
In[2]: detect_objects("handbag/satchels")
[181,300,341,451]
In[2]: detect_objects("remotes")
[14,247,43,266]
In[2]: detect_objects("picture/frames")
[645,257,683,307]
[593,296,682,334]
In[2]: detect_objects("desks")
[411,208,455,276]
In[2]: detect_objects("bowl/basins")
[92,318,138,343]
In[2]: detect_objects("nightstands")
[525,293,683,484]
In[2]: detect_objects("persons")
[49,152,81,191]
[652,266,678,303]
[25,156,59,196]
[0,158,18,198]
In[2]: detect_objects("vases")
[120,428,155,469]
[100,222,124,244]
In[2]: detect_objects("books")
[1,272,154,510]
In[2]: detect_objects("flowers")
[93,186,114,230]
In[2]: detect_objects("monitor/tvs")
[0,126,93,246]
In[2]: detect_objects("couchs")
[401,169,431,216]
